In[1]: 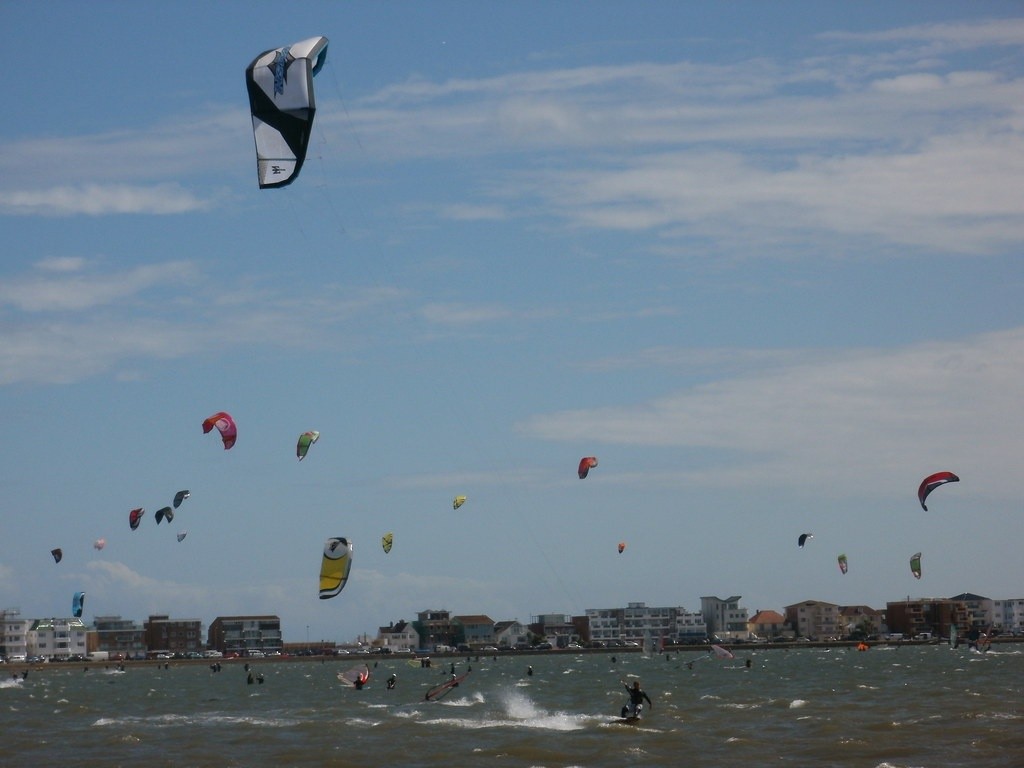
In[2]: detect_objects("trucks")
[592,642,605,648]
[607,643,620,647]
[441,646,457,652]
[557,638,578,649]
[200,650,217,657]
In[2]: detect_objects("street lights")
[306,626,310,651]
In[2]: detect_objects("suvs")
[865,634,881,641]
[623,641,639,647]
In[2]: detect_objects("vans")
[886,634,903,641]
[998,633,1013,638]
[357,650,370,655]
[568,644,581,648]
[915,633,931,640]
[210,652,223,658]
[86,652,109,661]
[9,656,24,663]
[337,650,350,655]
[248,650,264,657]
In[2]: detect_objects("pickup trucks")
[268,651,281,656]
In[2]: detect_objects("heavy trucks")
[713,631,758,643]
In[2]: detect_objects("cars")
[129,656,146,660]
[398,648,410,653]
[517,644,533,650]
[484,646,498,651]
[152,649,204,659]
[499,645,516,650]
[732,636,787,643]
[536,643,553,650]
[370,648,380,653]
[110,654,125,661]
[797,638,809,642]
[804,635,818,641]
[658,638,710,645]
[824,636,837,641]
[28,654,83,662]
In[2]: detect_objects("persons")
[528,666,532,675]
[450,663,455,674]
[244,664,251,672]
[256,673,264,684]
[621,680,652,718]
[387,673,396,689]
[421,657,431,668]
[248,673,253,684]
[450,674,459,687]
[354,676,363,690]
[214,662,222,672]
